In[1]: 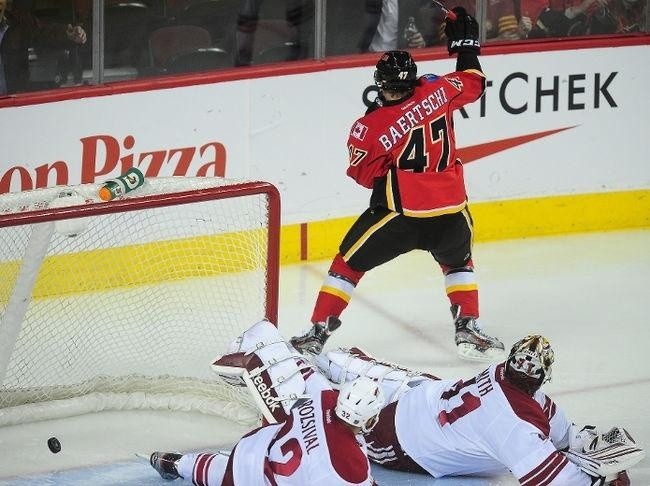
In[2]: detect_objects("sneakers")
[149,451,182,480]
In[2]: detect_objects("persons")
[435,0,521,43]
[518,0,598,38]
[593,0,650,36]
[355,0,427,55]
[233,0,308,67]
[0,0,89,94]
[287,4,510,353]
[148,318,645,484]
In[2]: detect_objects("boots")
[289,315,342,356]
[449,304,505,353]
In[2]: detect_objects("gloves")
[444,6,482,56]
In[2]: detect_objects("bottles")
[404,16,418,43]
[99,167,145,201]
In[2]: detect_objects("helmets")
[334,377,385,434]
[373,49,418,96]
[505,333,555,393]
